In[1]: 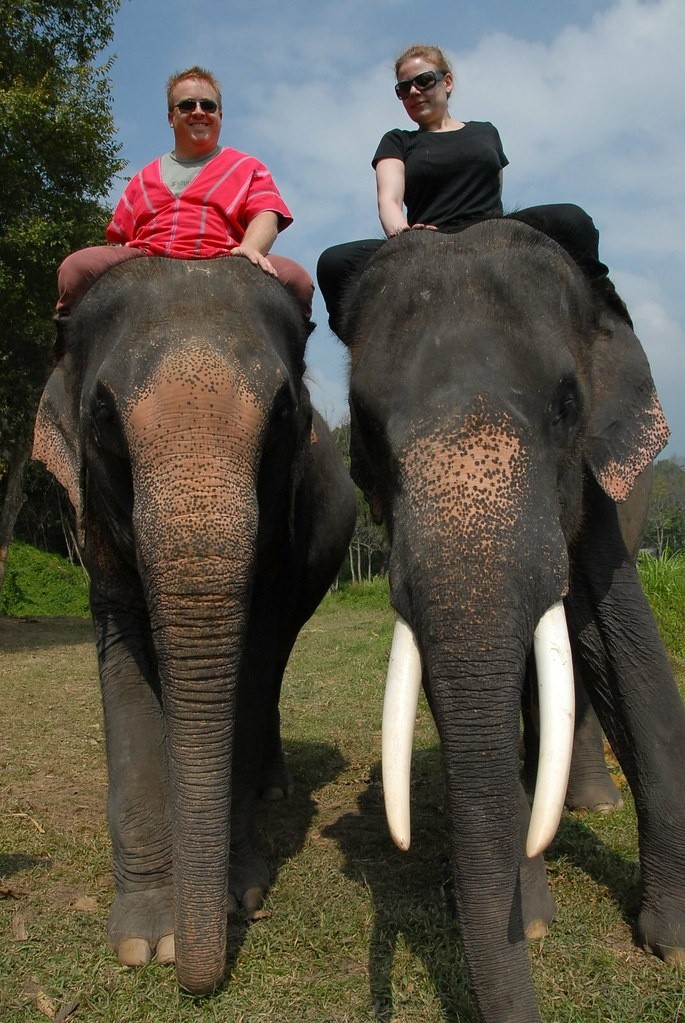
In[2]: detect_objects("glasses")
[170,99,219,114]
[394,71,444,100]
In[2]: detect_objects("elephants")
[316,201,685,1023]
[19,254,359,993]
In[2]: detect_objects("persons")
[50,70,314,369]
[317,46,600,345]
